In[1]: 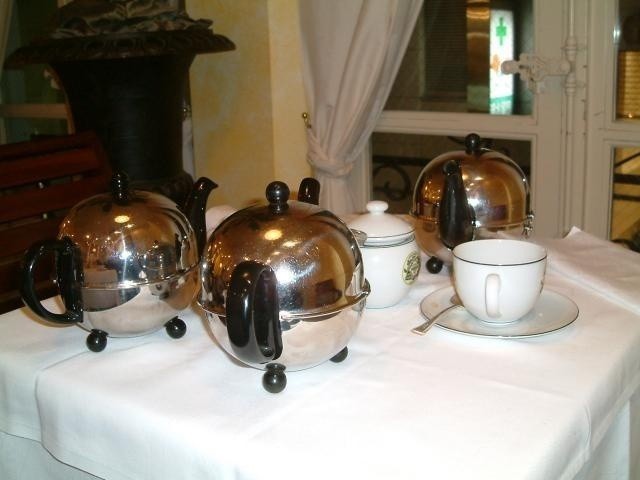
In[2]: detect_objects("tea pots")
[409,134,533,275]
[19,168,219,352]
[198,178,371,392]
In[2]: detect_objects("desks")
[0,234,639,480]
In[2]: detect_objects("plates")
[415,282,581,339]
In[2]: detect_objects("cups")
[347,199,422,308]
[452,239,548,327]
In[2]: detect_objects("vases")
[0,31,238,211]
